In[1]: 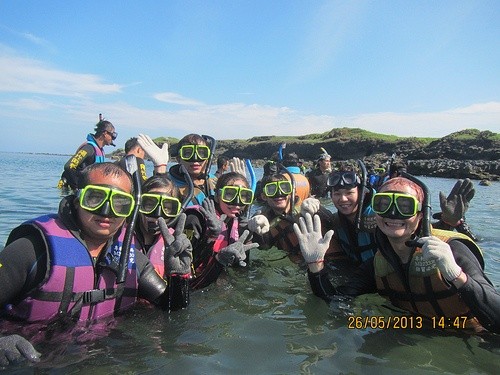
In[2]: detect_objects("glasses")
[138,192,182,219]
[72,184,136,219]
[179,143,211,162]
[325,172,362,189]
[371,192,419,219]
[262,180,293,198]
[217,185,255,206]
[101,130,118,141]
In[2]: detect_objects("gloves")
[228,156,247,179]
[0,332,43,371]
[136,132,170,167]
[299,197,321,217]
[197,197,222,237]
[439,177,476,226]
[293,212,335,265]
[416,235,462,283]
[217,228,260,269]
[247,215,271,235]
[157,213,193,275]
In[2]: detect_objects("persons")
[247,173,344,271]
[253,161,280,202]
[213,156,230,180]
[0,162,193,371]
[292,170,500,339]
[133,171,259,305]
[307,153,339,214]
[376,162,408,190]
[432,211,480,241]
[117,136,147,186]
[183,172,252,270]
[326,170,475,268]
[135,132,247,207]
[63,112,119,193]
